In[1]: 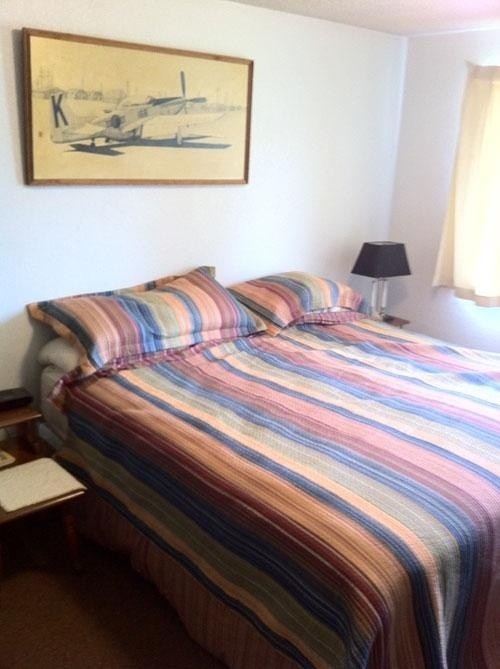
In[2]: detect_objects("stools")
[0,456,89,577]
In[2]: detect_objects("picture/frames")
[21,27,255,186]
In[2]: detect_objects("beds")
[25,320,499,668]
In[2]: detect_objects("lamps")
[350,240,411,321]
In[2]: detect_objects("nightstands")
[359,311,410,329]
[1,388,44,471]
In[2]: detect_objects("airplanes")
[49,69,208,153]
